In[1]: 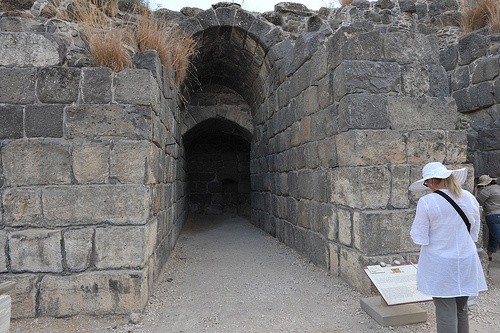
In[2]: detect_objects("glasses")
[423,182,429,187]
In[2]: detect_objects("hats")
[477,175,497,185]
[408,162,468,192]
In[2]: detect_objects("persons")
[408,162,489,333]
[475,175,500,261]
